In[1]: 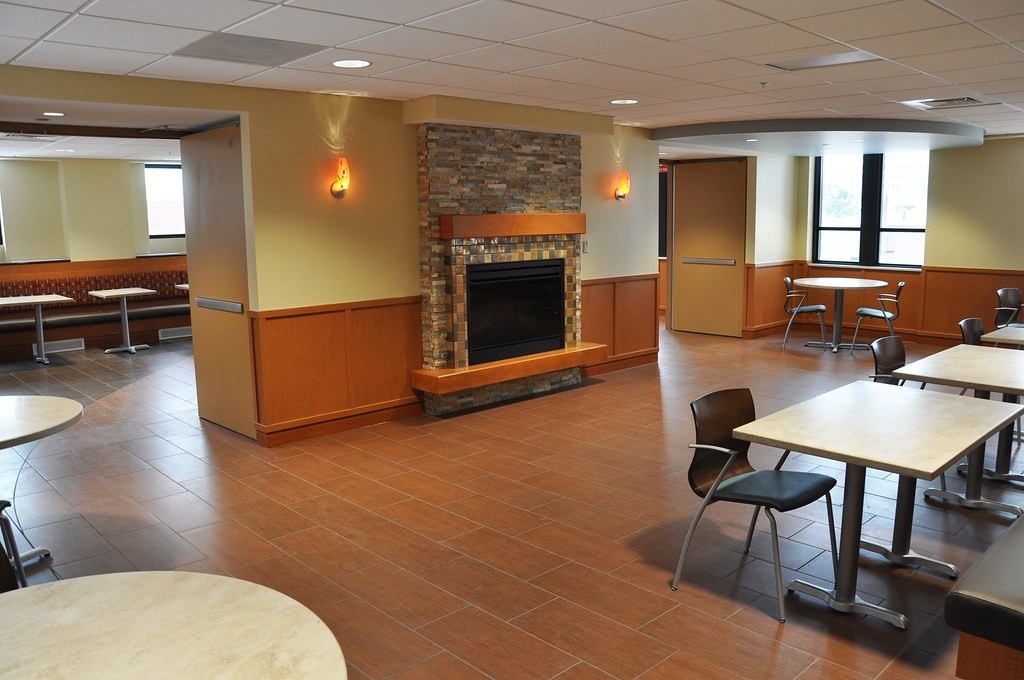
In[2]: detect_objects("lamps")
[616,172,630,200]
[331,158,350,198]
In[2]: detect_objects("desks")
[0,294,74,364]
[88,287,158,354]
[0,396,83,568]
[793,278,888,353]
[734,327,1024,630]
[0,570,349,680]
[175,284,189,290]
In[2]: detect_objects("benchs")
[945,515,1024,680]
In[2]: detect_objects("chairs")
[958,318,984,395]
[850,282,905,355]
[671,389,839,623]
[870,336,926,390]
[994,288,1024,350]
[783,276,827,351]
